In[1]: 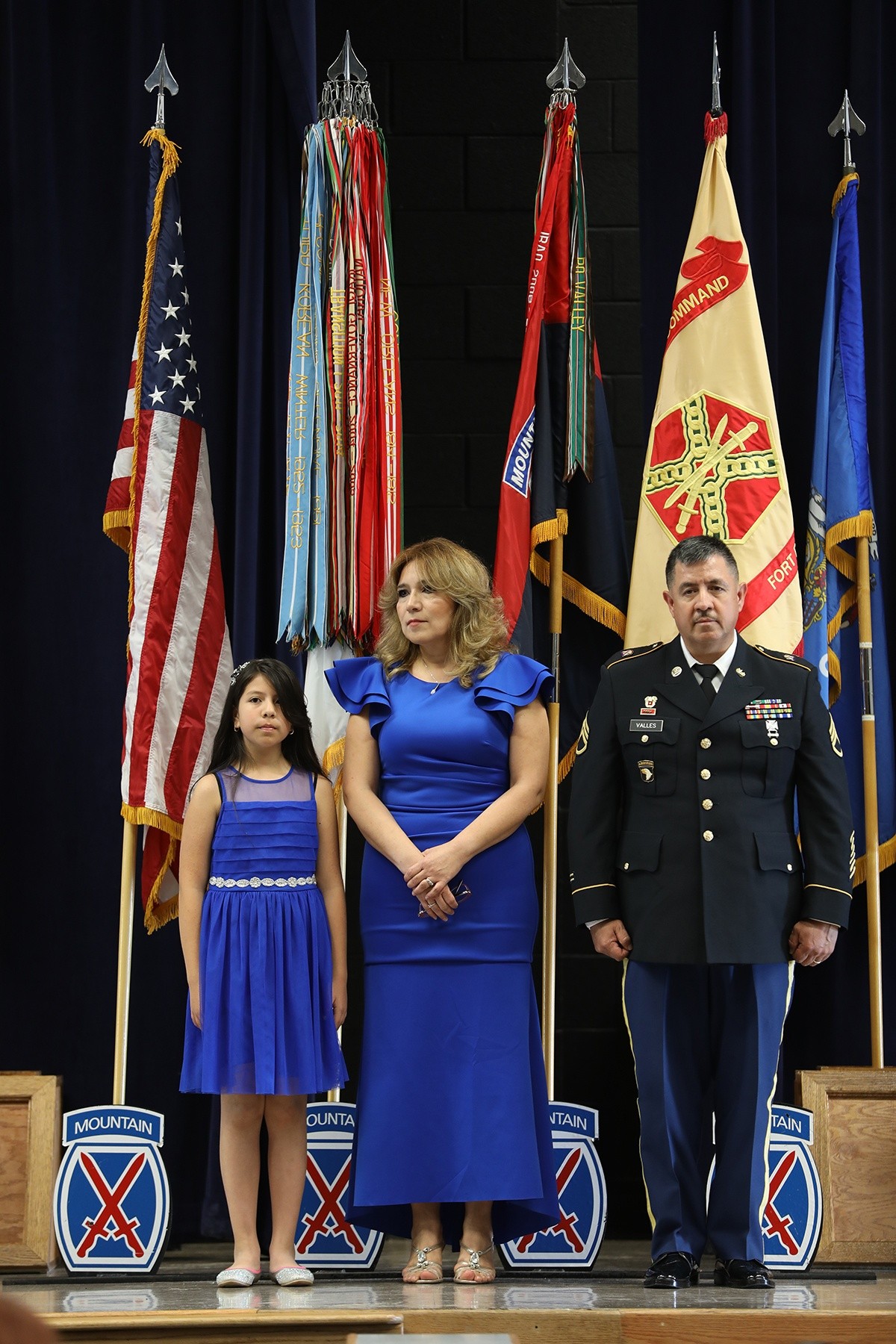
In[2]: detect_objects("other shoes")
[271,1266,314,1284]
[217,1268,261,1287]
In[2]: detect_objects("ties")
[692,663,720,706]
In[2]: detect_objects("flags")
[275,117,411,830]
[624,108,805,661]
[488,92,627,814]
[103,126,237,937]
[793,174,896,893]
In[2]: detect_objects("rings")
[814,960,821,964]
[428,902,436,908]
[426,878,435,886]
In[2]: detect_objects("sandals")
[403,1243,444,1283]
[453,1241,495,1283]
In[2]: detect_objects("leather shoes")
[714,1260,776,1288]
[642,1252,700,1289]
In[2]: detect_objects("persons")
[323,538,561,1284]
[178,658,348,1287]
[568,535,857,1287]
